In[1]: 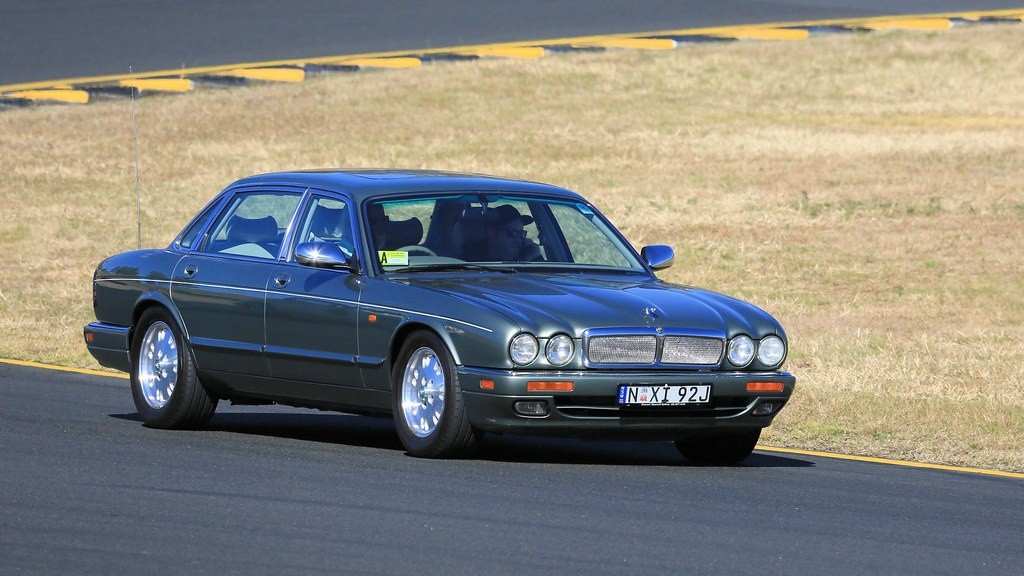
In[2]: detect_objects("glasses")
[505,230,527,240]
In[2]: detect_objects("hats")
[487,204,534,228]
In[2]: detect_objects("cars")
[83,169,798,466]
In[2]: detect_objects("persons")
[468,205,544,262]
[295,203,386,266]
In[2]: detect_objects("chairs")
[225,214,281,242]
[449,217,487,261]
[386,216,423,251]
